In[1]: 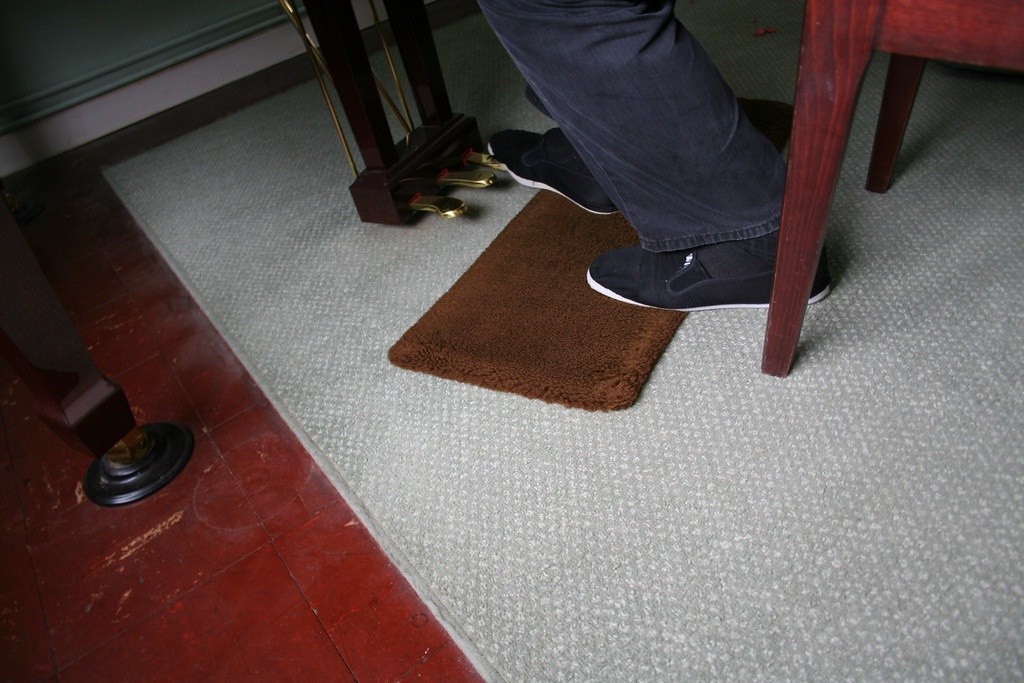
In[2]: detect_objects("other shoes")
[587,243,833,312]
[488,129,622,217]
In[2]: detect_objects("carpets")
[104,0,1024,683]
[387,86,794,412]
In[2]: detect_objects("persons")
[478,1,832,313]
